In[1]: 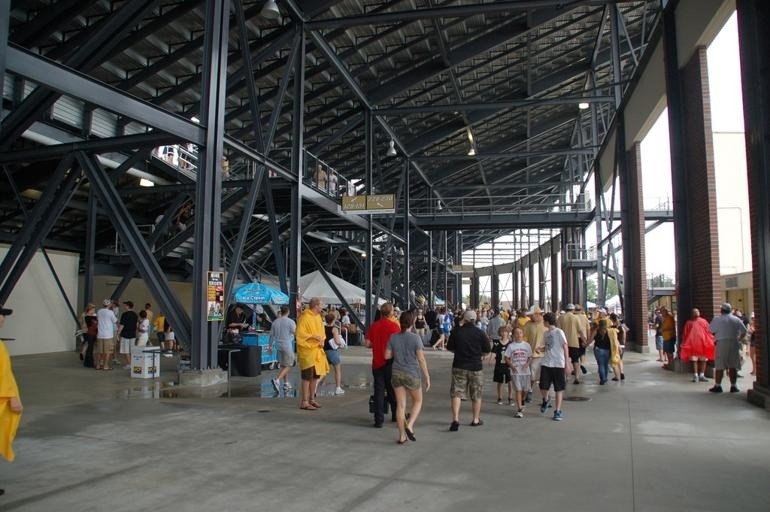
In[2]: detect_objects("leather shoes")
[709,386,722,393]
[730,387,739,392]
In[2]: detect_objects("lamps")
[386,137,398,157]
[579,93,590,110]
[140,177,155,187]
[259,0,280,19]
[435,199,442,210]
[468,144,476,157]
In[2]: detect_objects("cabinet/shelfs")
[242,329,295,364]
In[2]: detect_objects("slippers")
[300,405,316,410]
[471,417,484,426]
[580,365,587,374]
[96,368,102,370]
[104,368,114,370]
[573,380,580,384]
[449,421,459,431]
[399,437,407,444]
[405,428,416,441]
[310,402,321,408]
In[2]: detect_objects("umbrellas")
[230,282,289,327]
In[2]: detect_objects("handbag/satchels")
[369,395,390,414]
[328,335,345,350]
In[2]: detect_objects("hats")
[720,303,731,311]
[123,301,134,309]
[527,304,544,315]
[103,299,113,306]
[566,304,575,310]
[596,307,607,315]
[464,311,477,323]
[0,306,13,316]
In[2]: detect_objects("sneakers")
[335,387,345,395]
[123,364,131,370]
[699,376,709,382]
[79,352,84,361]
[612,373,624,381]
[271,378,280,392]
[497,398,503,405]
[693,376,698,382]
[540,396,551,413]
[509,398,514,406]
[554,410,563,421]
[515,409,523,418]
[163,351,174,358]
[283,383,293,390]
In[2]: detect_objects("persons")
[313,164,327,188]
[364,303,630,444]
[224,297,351,410]
[0,303,24,496]
[74,298,183,371]
[652,302,756,393]
[222,159,230,178]
[326,169,337,193]
[157,116,198,169]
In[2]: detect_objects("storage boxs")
[131,345,160,379]
